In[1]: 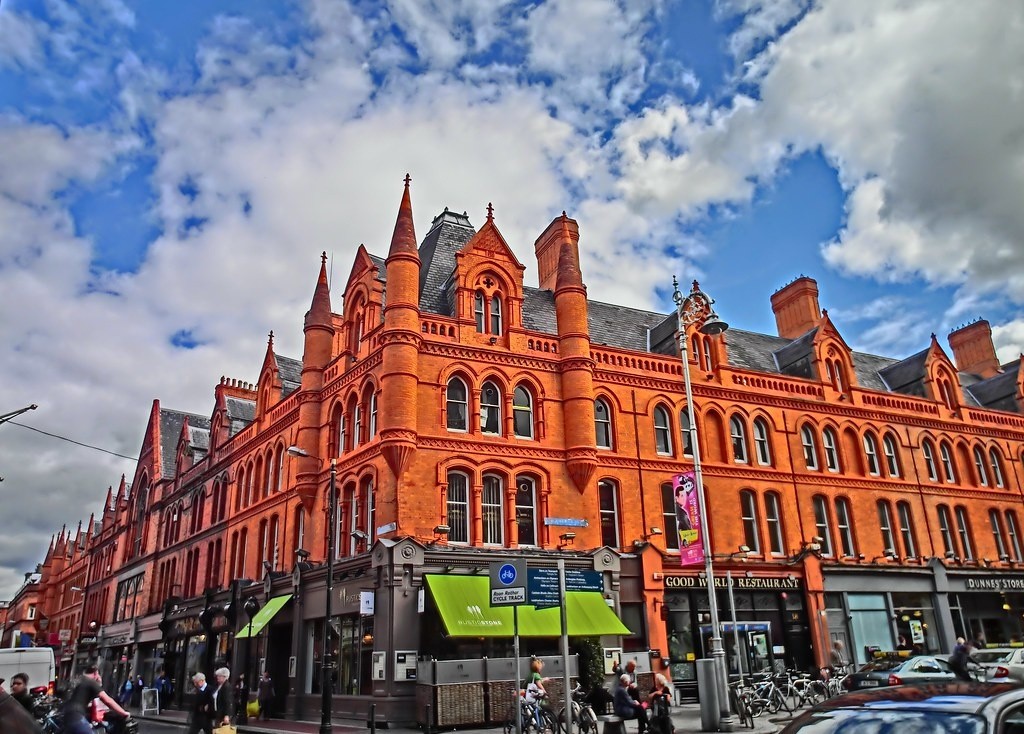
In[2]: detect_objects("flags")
[21,633,31,647]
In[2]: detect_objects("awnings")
[423,574,631,640]
[233,594,293,639]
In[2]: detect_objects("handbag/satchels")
[525,673,538,703]
[211,721,236,734]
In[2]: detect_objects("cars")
[846,648,1024,692]
[778,680,1024,734]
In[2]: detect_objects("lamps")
[294,548,322,565]
[803,536,824,545]
[642,526,663,541]
[557,532,577,550]
[427,525,452,545]
[287,446,326,467]
[983,553,1009,563]
[938,551,955,560]
[872,548,894,563]
[729,545,751,556]
[349,530,371,546]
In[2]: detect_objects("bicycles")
[559,682,598,734]
[728,663,855,729]
[504,690,561,734]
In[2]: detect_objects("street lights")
[671,275,734,733]
[232,595,260,725]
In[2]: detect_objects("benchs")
[597,711,653,734]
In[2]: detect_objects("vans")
[0,647,55,697]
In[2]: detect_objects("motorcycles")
[29,686,139,734]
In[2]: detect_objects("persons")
[256,669,275,720]
[607,660,676,734]
[897,636,921,657]
[683,539,689,548]
[755,638,763,655]
[188,667,235,734]
[952,632,987,681]
[154,669,171,711]
[0,673,45,734]
[675,486,692,531]
[63,663,131,734]
[522,658,552,731]
[119,671,144,710]
[815,640,848,700]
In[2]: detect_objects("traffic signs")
[527,567,604,603]
